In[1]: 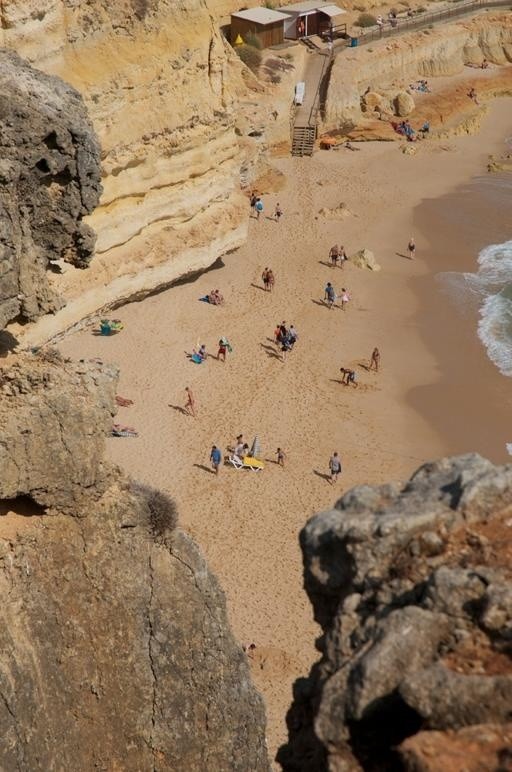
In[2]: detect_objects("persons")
[215,289,225,303]
[261,267,269,291]
[367,347,381,373]
[469,88,480,107]
[407,237,416,259]
[184,386,196,417]
[209,290,222,306]
[396,117,430,141]
[480,59,491,70]
[336,287,351,309]
[275,448,287,466]
[274,321,298,361]
[254,198,263,220]
[250,192,256,215]
[324,282,336,309]
[200,345,208,360]
[113,424,136,435]
[338,246,347,270]
[209,445,224,476]
[234,433,244,448]
[267,270,276,293]
[216,339,227,363]
[329,244,340,267]
[339,367,358,390]
[328,451,342,485]
[376,15,385,27]
[235,442,249,458]
[419,80,431,93]
[274,202,282,222]
[116,396,134,407]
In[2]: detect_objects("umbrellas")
[250,434,262,459]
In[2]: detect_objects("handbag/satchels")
[282,345,288,351]
[290,338,296,344]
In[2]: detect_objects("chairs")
[229,455,264,472]
